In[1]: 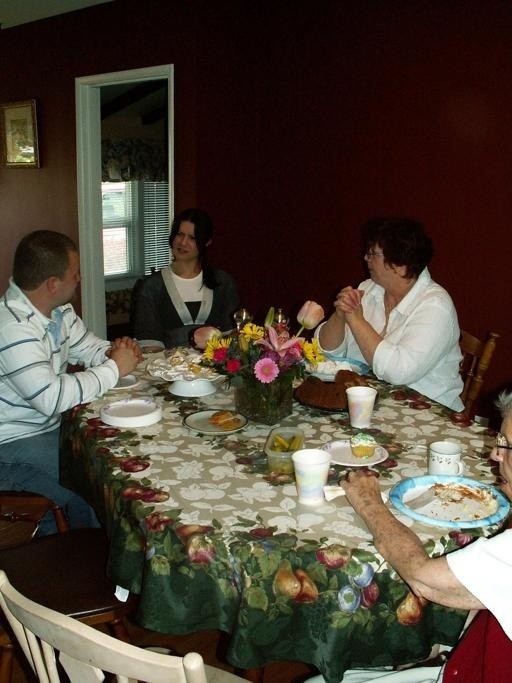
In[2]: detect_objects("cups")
[346,386,377,429]
[290,447,331,505]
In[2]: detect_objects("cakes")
[165,346,187,364]
[349,432,377,457]
[293,370,362,412]
[220,418,239,429]
[209,410,232,425]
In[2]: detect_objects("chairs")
[459,328,500,417]
[0,490,135,683]
[0,568,253,683]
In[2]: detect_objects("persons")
[0,229,142,537]
[303,390,512,683]
[311,218,467,414]
[129,207,240,349]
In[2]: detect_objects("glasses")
[366,251,382,258]
[495,434,512,453]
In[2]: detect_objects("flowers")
[197,301,326,426]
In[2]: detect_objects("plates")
[321,438,390,467]
[110,373,141,391]
[100,398,162,428]
[182,408,248,436]
[139,339,165,357]
[388,474,509,529]
[313,355,370,382]
[427,440,463,475]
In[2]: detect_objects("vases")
[234,373,293,424]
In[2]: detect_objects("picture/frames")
[0,99,42,170]
